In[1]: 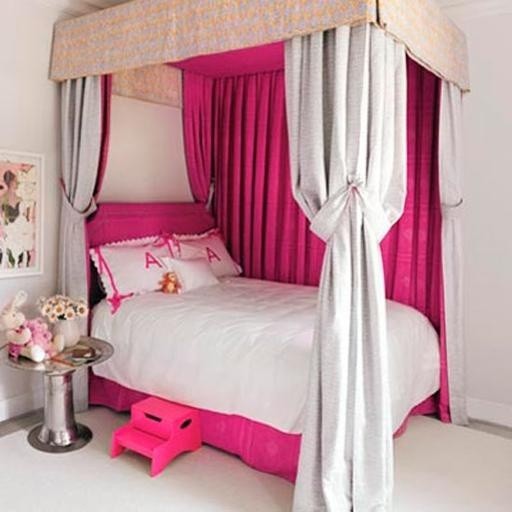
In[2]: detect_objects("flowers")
[38,294,87,321]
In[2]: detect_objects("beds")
[48,1,473,512]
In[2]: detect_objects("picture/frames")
[0,150,45,279]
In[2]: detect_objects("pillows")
[88,235,172,314]
[161,255,220,294]
[163,227,244,278]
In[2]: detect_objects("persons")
[0,171,32,267]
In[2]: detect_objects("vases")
[52,317,81,348]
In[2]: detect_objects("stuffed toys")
[158,272,182,294]
[0,290,66,362]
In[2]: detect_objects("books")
[52,341,103,367]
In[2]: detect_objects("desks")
[0,337,113,453]
